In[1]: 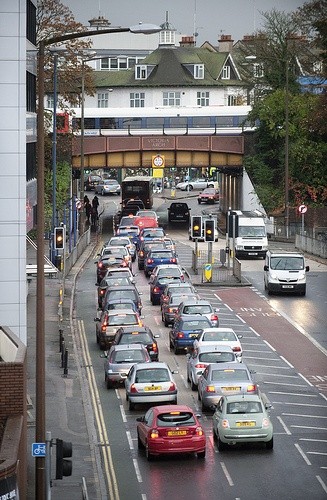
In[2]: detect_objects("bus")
[122,175,154,209]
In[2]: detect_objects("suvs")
[107,326,160,362]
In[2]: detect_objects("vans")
[264,247,310,297]
[225,210,268,259]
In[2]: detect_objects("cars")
[209,394,274,452]
[136,405,206,462]
[84,175,243,363]
[197,362,259,414]
[186,345,239,391]
[121,362,179,411]
[100,345,152,390]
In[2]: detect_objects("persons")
[84,202,91,221]
[92,196,99,212]
[90,207,99,225]
[84,195,90,203]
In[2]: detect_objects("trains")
[43,106,260,136]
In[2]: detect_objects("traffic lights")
[73,168,82,179]
[54,227,64,249]
[204,219,215,242]
[56,438,73,480]
[191,216,202,239]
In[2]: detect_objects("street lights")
[245,54,289,239]
[79,55,128,233]
[33,22,163,500]
